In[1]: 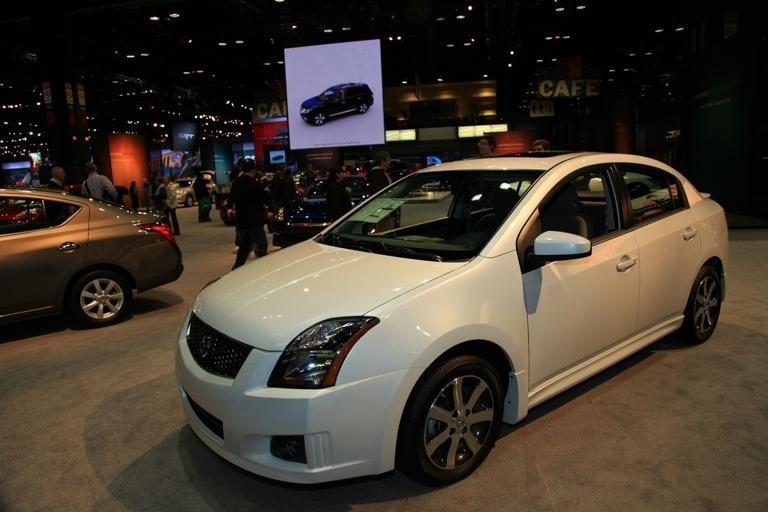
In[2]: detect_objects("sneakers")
[171,232,181,235]
[232,246,240,253]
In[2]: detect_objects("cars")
[161,163,395,249]
[1,180,190,339]
[168,144,736,496]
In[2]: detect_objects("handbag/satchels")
[198,197,212,215]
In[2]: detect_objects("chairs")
[472,190,517,232]
[540,184,587,238]
[627,181,650,200]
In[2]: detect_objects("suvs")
[298,81,376,128]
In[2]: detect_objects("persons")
[476,135,498,157]
[44,166,74,225]
[193,170,212,223]
[225,151,394,269]
[128,175,181,236]
[80,161,119,201]
[532,138,551,151]
